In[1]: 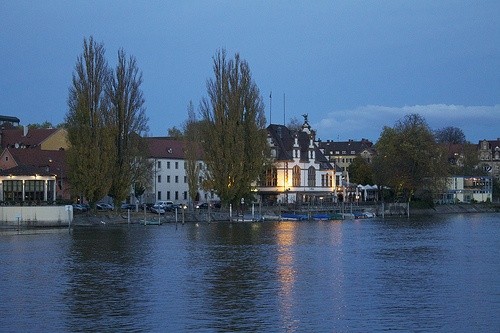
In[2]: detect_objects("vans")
[154,200,173,209]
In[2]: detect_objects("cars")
[152,206,165,214]
[195,202,215,209]
[71,200,153,210]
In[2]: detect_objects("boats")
[230,209,377,224]
